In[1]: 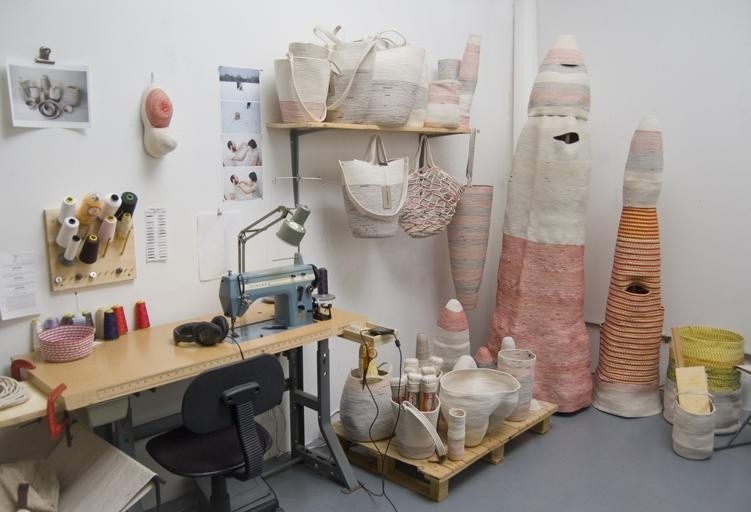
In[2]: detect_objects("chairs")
[145,353,285,512]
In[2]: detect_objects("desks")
[0,297,398,512]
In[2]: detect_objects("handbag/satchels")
[339,135,409,239]
[399,135,463,238]
[273,24,463,127]
[670,390,716,460]
[446,128,493,313]
[388,393,446,461]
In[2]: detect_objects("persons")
[236,172,263,199]
[226,174,249,200]
[224,141,250,166]
[248,139,262,166]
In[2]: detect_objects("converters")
[370,327,394,335]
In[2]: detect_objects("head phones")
[173,315,229,346]
